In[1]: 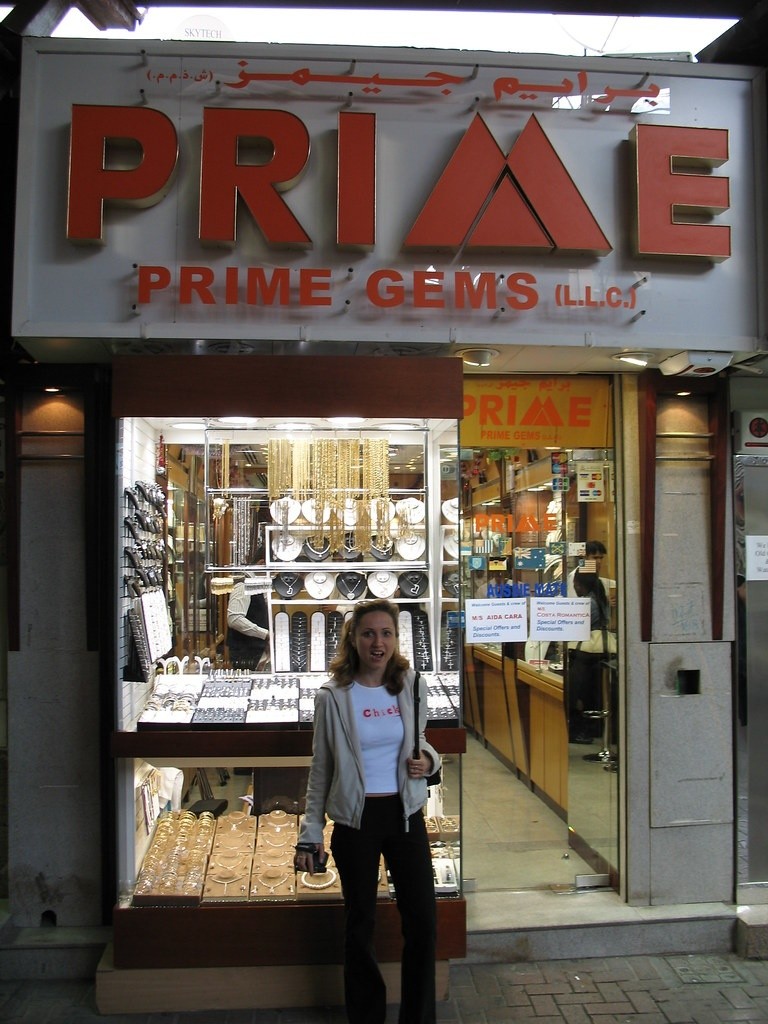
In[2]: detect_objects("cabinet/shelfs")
[466,455,619,826]
[96,348,470,968]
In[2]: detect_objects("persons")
[225,547,275,672]
[293,602,442,1023]
[566,540,616,740]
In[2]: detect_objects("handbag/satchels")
[413,671,441,787]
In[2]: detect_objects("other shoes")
[569,735,592,744]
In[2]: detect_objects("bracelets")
[136,809,215,896]
[138,667,458,724]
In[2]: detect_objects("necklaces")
[211,437,459,672]
[212,814,460,894]
[124,484,168,596]
